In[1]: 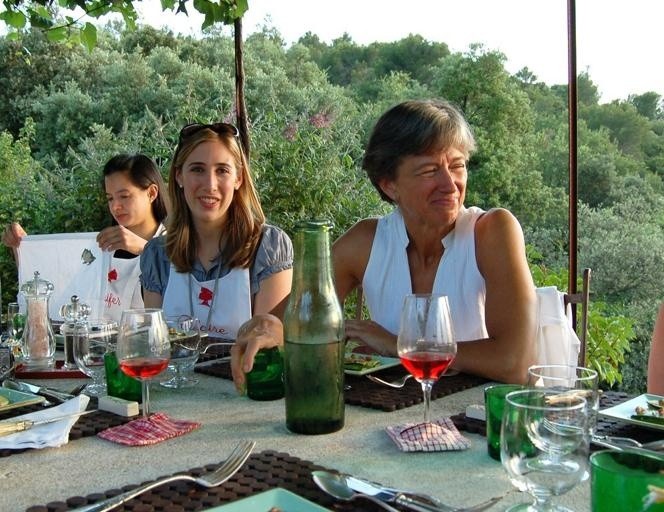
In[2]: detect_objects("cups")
[102,351,144,404]
[483,386,541,462]
[6,302,28,354]
[588,441,664,511]
[238,345,284,401]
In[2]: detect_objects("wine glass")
[161,314,199,390]
[70,318,114,394]
[112,308,175,433]
[396,293,458,445]
[497,389,592,512]
[524,364,606,476]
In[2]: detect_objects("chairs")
[564,268,590,390]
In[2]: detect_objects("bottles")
[281,220,346,436]
[18,269,57,365]
[56,295,91,370]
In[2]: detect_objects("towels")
[0,394,91,450]
[383,414,474,454]
[98,413,201,448]
[17,232,110,323]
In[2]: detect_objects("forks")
[381,496,507,511]
[364,367,458,388]
[174,335,237,356]
[68,437,257,512]
[18,379,87,402]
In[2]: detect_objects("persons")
[230,97,540,397]
[1,155,169,322]
[140,122,297,340]
[647,295,663,394]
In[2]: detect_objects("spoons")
[594,432,664,452]
[310,466,406,511]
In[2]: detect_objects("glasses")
[180,123,243,163]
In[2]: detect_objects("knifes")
[342,473,447,512]
[0,408,94,439]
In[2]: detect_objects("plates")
[596,390,663,428]
[0,387,46,413]
[202,486,334,512]
[341,349,399,379]
